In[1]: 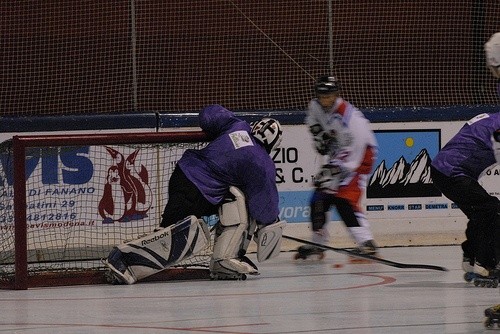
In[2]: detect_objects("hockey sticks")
[253,231,449,272]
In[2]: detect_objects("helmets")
[315,76,338,94]
[251,118,282,154]
[484,32,500,67]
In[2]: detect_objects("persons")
[484,32,500,81]
[153,104,287,282]
[293,74,380,262]
[430,109,500,288]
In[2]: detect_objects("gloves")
[314,163,344,191]
[310,123,337,155]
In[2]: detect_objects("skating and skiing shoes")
[104,268,123,285]
[209,256,261,281]
[348,227,378,260]
[484,303,500,329]
[295,229,327,260]
[473,257,500,288]
[462,253,475,282]
